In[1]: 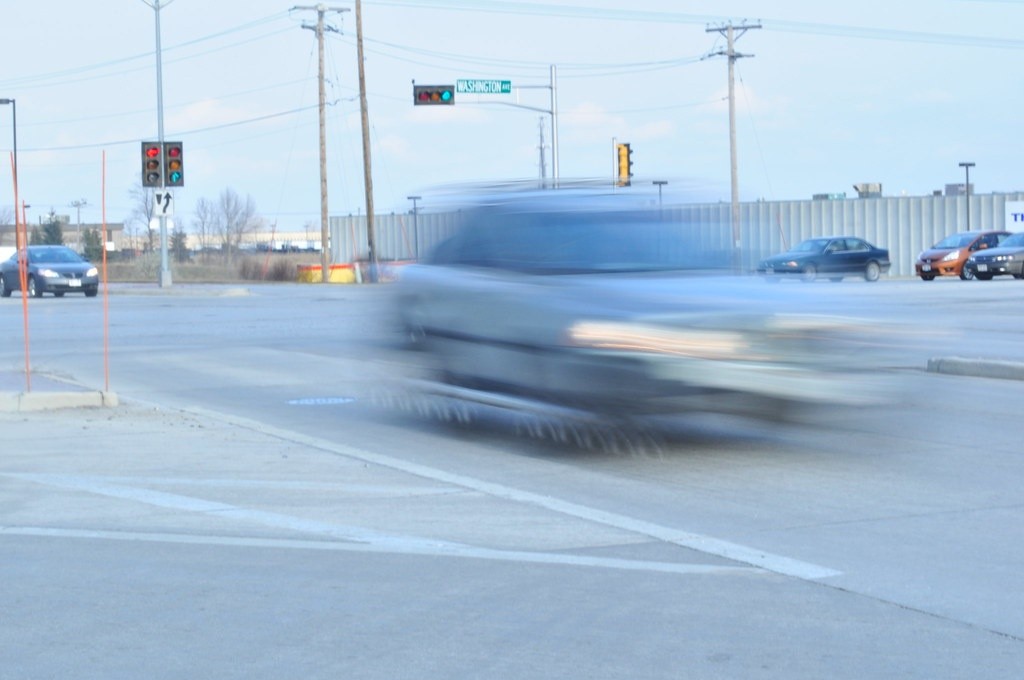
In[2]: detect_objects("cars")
[915,230,1015,281]
[756,235,891,283]
[390,188,880,452]
[0,245,100,299]
[965,232,1023,281]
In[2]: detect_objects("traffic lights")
[414,85,456,106]
[141,142,162,188]
[162,142,186,187]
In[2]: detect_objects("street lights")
[0,97,21,250]
[959,161,976,234]
[406,195,423,263]
